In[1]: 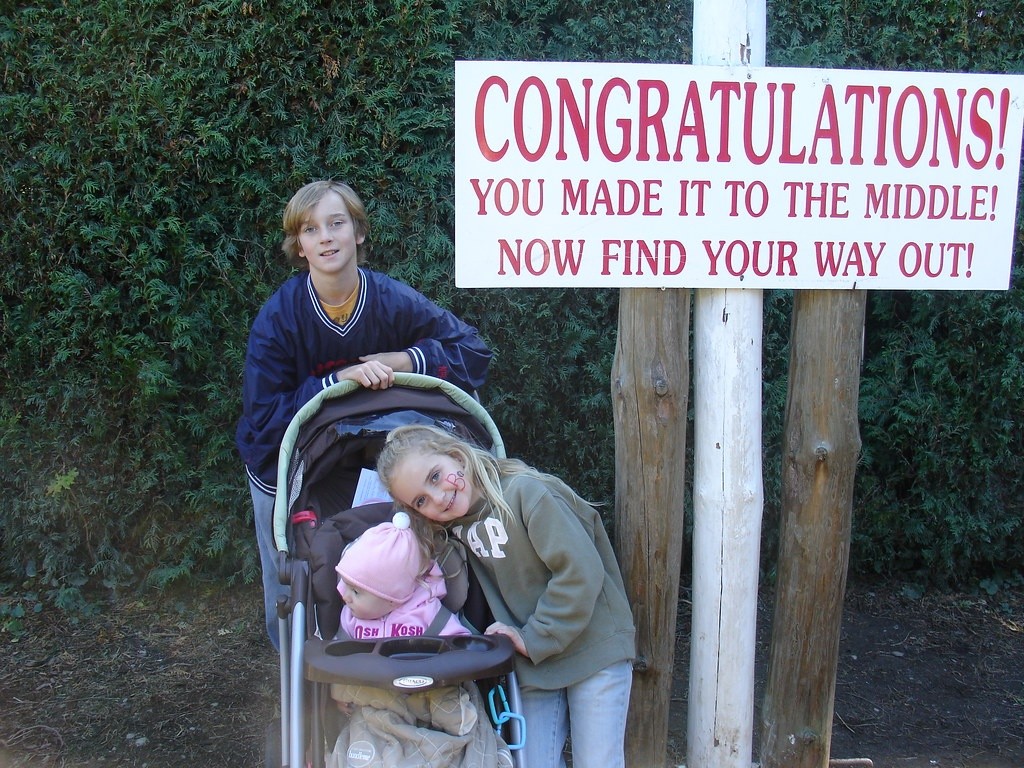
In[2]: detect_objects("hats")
[335,512,421,604]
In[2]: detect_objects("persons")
[234,181,493,653]
[376,424,636,768]
[325,511,513,768]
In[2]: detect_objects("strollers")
[270,370,529,768]
